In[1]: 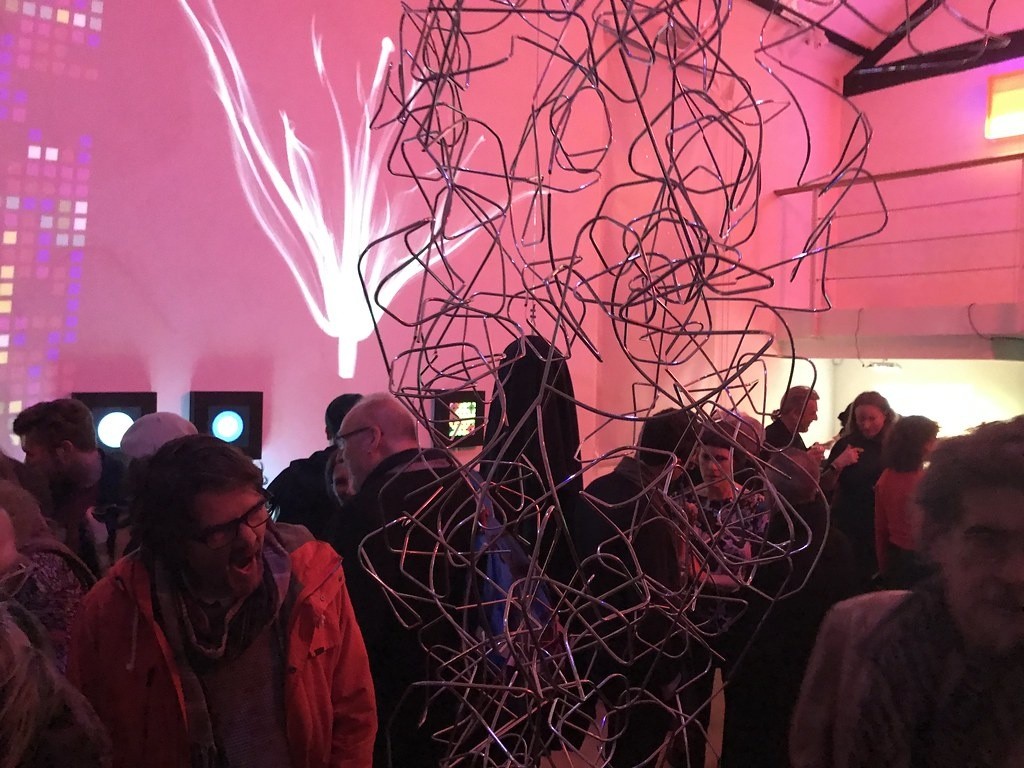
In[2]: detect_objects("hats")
[120,411,199,460]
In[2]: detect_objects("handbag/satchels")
[481,334,587,640]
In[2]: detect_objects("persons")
[0,385,1023,768]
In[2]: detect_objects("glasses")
[182,487,276,551]
[333,425,372,450]
